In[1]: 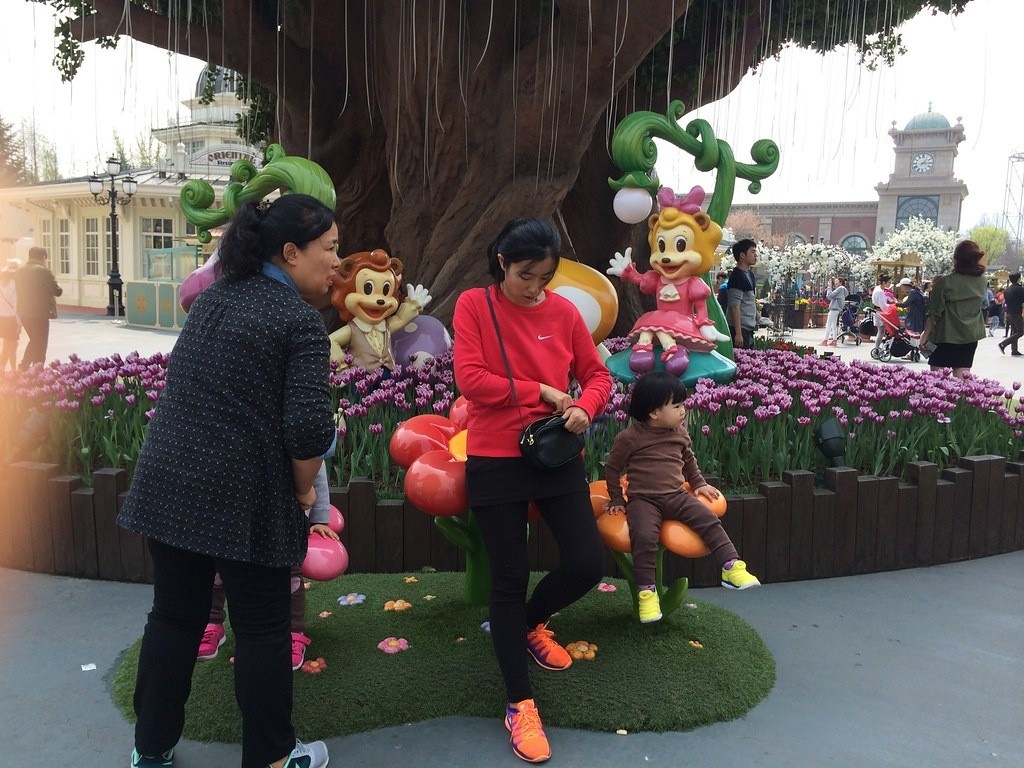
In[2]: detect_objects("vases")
[803,310,811,327]
[814,312,828,328]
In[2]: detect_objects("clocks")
[912,153,934,174]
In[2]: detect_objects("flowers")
[797,298,809,307]
[814,300,829,312]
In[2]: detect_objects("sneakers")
[636,586,663,624]
[526,618,572,670]
[195,624,226,661]
[130,744,174,768]
[290,631,312,670]
[504,699,552,762]
[265,738,329,768]
[720,560,762,591]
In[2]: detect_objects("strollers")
[837,294,864,346]
[865,307,923,362]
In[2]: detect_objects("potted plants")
[848,301,874,340]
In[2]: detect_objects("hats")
[895,277,911,287]
[1,258,23,272]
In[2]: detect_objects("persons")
[982,281,1006,328]
[919,240,987,382]
[894,278,926,360]
[921,279,931,296]
[726,239,774,350]
[997,273,1024,355]
[777,277,800,328]
[817,277,849,346]
[605,371,761,622]
[872,273,899,355]
[114,192,336,768]
[0,245,63,374]
[453,216,614,764]
[717,272,729,315]
[933,274,943,285]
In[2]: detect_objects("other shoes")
[901,354,912,360]
[817,340,827,346]
[988,330,993,337]
[829,340,837,346]
[1011,351,1024,355]
[999,343,1005,354]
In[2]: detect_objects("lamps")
[813,417,847,468]
[11,406,50,462]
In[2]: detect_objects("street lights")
[88,152,138,317]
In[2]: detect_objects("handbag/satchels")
[48,292,57,319]
[860,311,878,336]
[519,414,587,472]
